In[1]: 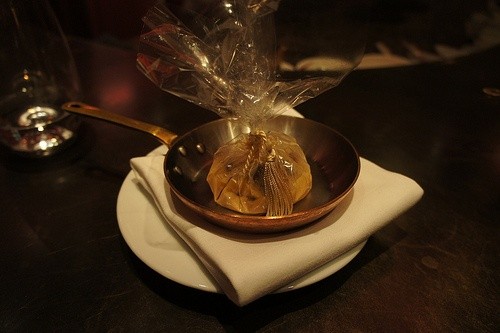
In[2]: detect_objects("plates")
[116,143,369,294]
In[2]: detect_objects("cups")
[0,0,78,152]
